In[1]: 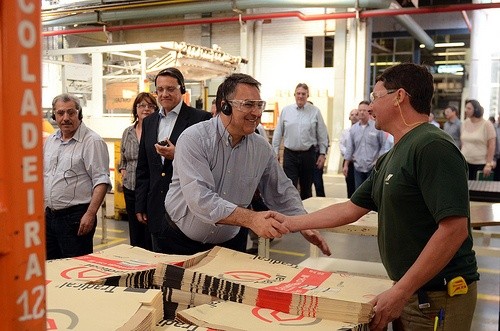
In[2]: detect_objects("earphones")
[394,95,400,102]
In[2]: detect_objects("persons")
[135,67,213,254]
[460,99,496,180]
[44,94,112,260]
[272,83,329,201]
[340,100,394,199]
[428,112,440,128]
[158,73,332,256]
[119,92,159,252]
[488,116,500,181]
[265,63,480,331]
[443,106,462,151]
[211,99,274,248]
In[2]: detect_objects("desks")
[470,202,500,331]
[264,196,379,261]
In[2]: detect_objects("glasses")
[137,102,157,110]
[369,89,412,103]
[228,99,266,113]
[52,108,79,118]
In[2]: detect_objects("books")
[477,171,494,181]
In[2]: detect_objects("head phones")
[51,95,84,121]
[156,69,186,94]
[222,80,232,115]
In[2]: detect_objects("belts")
[413,274,473,309]
[46,203,89,215]
